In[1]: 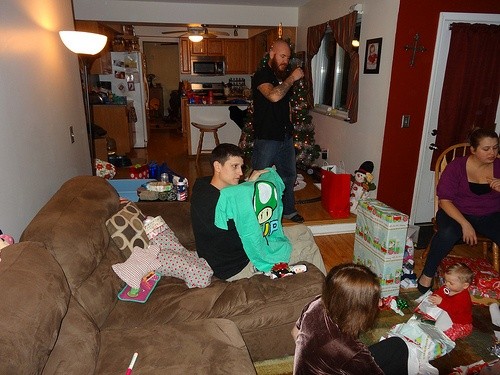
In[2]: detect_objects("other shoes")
[290,214,305,223]
[417,271,433,293]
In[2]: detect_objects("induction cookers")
[191,82,225,95]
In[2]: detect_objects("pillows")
[105,200,149,258]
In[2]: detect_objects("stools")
[191,122,227,163]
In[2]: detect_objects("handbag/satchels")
[320,159,352,218]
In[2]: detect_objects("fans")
[161,27,230,39]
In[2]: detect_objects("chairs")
[422,143,500,272]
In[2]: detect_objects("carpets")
[254,289,481,375]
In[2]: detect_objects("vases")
[149,77,154,87]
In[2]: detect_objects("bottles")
[181,80,189,92]
[228,77,245,92]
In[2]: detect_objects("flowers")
[147,73,156,78]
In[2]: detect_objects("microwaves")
[190,54,226,76]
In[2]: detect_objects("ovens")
[212,95,225,103]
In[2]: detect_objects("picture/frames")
[363,37,382,74]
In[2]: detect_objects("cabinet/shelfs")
[179,27,295,137]
[75,21,138,163]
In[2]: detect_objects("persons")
[290,262,409,375]
[191,143,327,282]
[428,262,473,341]
[252,40,304,223]
[418,130,500,292]
[368,45,377,65]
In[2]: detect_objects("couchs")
[0,176,325,375]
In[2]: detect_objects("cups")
[160,173,169,185]
[186,91,213,105]
[176,182,185,201]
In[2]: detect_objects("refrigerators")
[99,51,150,148]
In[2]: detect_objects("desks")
[149,86,163,103]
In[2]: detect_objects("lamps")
[189,31,203,42]
[58,30,108,176]
[233,25,240,36]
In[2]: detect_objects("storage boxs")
[108,179,155,202]
[128,163,150,180]
[353,199,408,299]
